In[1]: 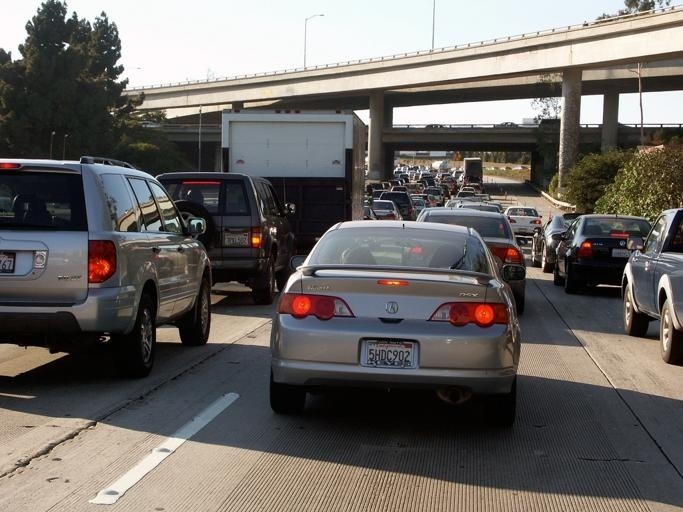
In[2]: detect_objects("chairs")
[342,246,376,265]
[425,243,469,271]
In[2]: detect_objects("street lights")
[304,13,325,68]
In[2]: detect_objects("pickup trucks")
[619,207,683,365]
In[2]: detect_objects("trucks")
[220,107,370,254]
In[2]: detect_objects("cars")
[502,206,543,243]
[362,107,503,223]
[552,214,651,294]
[416,208,526,317]
[531,212,584,273]
[267,218,524,433]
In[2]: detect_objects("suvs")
[152,170,298,307]
[0,154,216,381]
